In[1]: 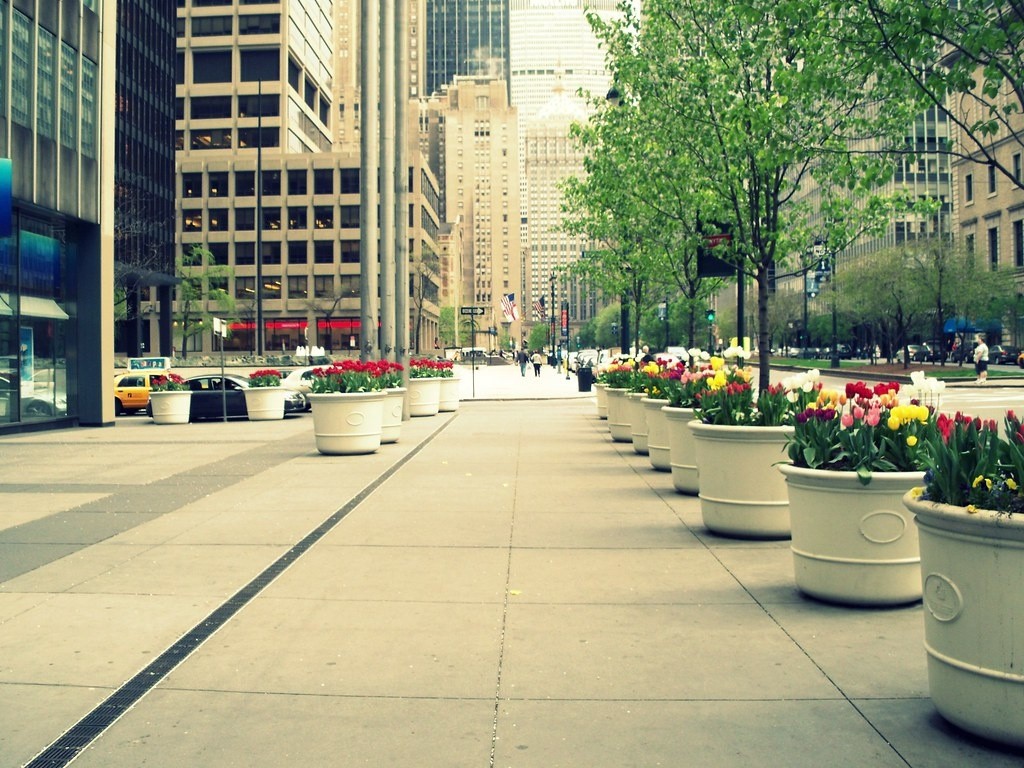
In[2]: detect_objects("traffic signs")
[460,307,485,315]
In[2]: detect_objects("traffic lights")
[704,309,716,321]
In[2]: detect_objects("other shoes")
[975,379,986,384]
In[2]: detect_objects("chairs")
[209,380,220,390]
[191,380,203,391]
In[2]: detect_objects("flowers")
[248,370,282,388]
[300,357,455,394]
[770,371,946,486]
[911,409,1024,514]
[692,365,830,427]
[592,357,740,408]
[152,373,190,392]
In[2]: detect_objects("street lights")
[813,240,840,368]
[548,276,557,369]
[605,84,631,364]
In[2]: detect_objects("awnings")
[0,293,69,320]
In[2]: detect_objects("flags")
[500,293,519,323]
[533,296,545,318]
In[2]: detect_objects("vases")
[640,397,672,471]
[307,391,388,455]
[438,378,459,412]
[777,460,926,605]
[604,388,634,442]
[903,486,1024,745]
[149,390,194,425]
[380,387,408,444]
[627,393,650,453]
[661,405,704,492]
[688,418,795,537]
[406,377,443,416]
[242,385,288,420]
[594,383,609,419]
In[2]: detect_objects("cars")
[113,370,209,416]
[0,366,54,397]
[775,339,853,361]
[146,373,307,422]
[0,375,69,418]
[1017,354,1024,370]
[987,344,1021,366]
[566,348,684,385]
[665,346,690,365]
[267,365,342,408]
[913,341,948,363]
[950,341,978,364]
[896,344,931,362]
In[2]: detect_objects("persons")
[515,347,529,376]
[640,345,655,363]
[974,338,989,384]
[530,349,544,377]
